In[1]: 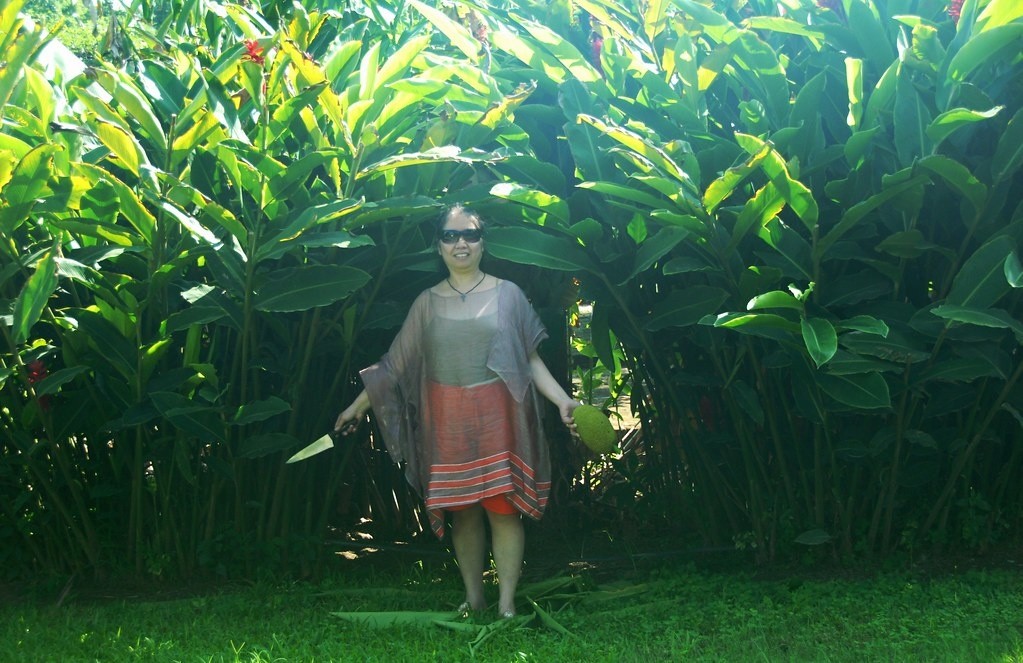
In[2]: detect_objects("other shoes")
[458,601,473,619]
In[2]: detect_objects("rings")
[566,424,569,428]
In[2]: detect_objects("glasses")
[439,228,481,243]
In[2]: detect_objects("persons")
[336,206,584,624]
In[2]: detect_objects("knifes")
[285,418,359,464]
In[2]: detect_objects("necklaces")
[447,271,485,301]
